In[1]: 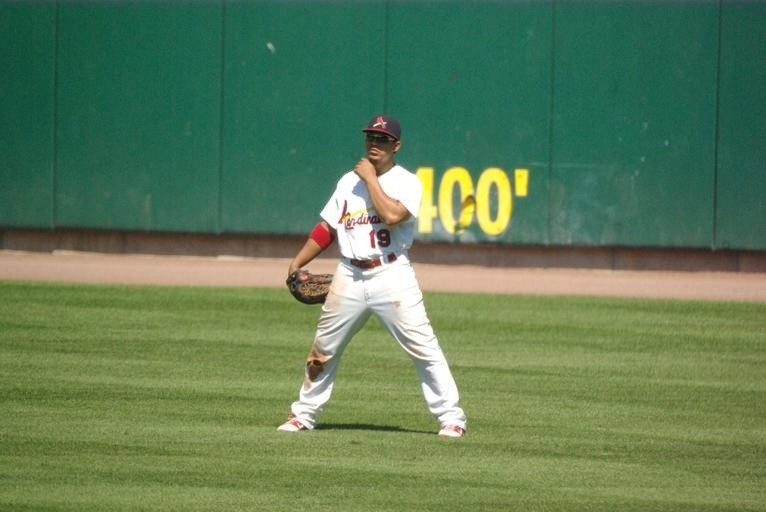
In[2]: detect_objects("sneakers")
[438,424,466,436]
[277,417,307,431]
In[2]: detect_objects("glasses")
[365,134,395,142]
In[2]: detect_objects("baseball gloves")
[287,269,334,303]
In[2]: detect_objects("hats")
[364,113,402,139]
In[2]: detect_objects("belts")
[343,254,396,268]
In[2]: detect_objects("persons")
[273,113,468,439]
